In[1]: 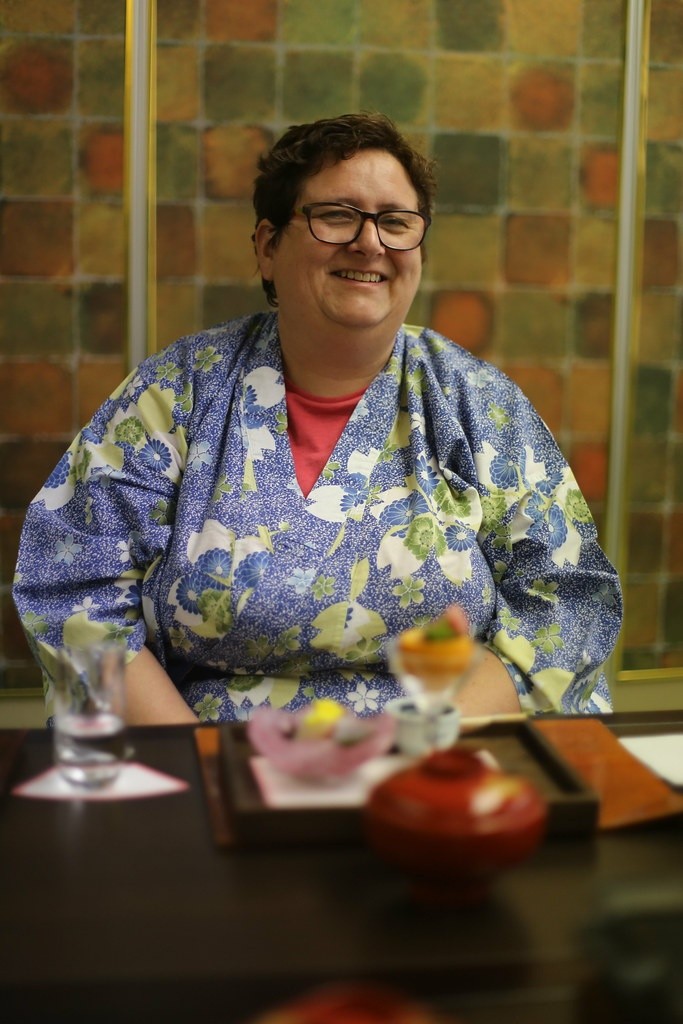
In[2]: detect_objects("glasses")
[302,202,431,251]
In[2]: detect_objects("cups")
[384,699,460,753]
[44,622,129,791]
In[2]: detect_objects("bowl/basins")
[250,704,392,779]
[365,749,551,897]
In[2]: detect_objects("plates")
[215,722,601,846]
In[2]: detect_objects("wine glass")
[388,635,483,755]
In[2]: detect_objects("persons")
[13,112,625,726]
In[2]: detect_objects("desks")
[0,710,683,1024]
